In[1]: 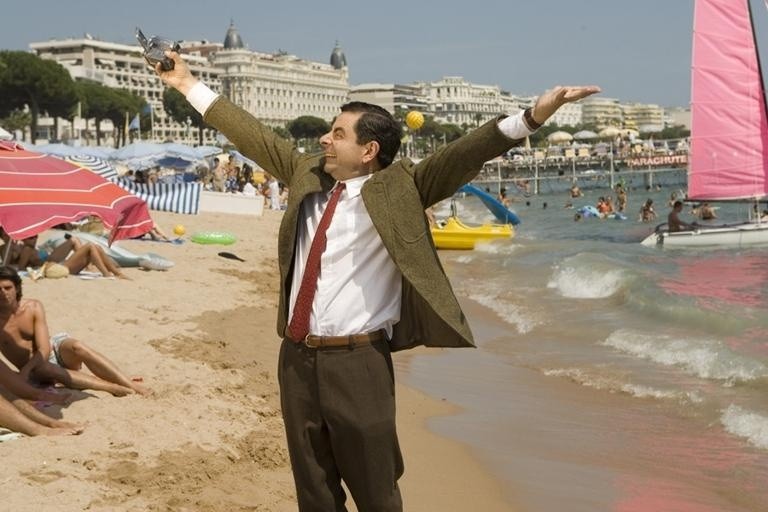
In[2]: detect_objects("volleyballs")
[405,111,424,130]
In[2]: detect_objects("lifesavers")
[189,230,237,246]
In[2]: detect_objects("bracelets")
[524,107,541,132]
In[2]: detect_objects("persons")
[1,226,178,435]
[127,157,290,211]
[424,131,768,232]
[153,50,602,512]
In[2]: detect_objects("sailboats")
[638,0,768,252]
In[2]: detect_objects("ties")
[290,182,345,344]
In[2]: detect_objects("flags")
[129,115,139,131]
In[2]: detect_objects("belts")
[285,327,385,348]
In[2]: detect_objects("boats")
[429,183,520,250]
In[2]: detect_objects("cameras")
[135,26,180,70]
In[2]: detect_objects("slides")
[457,183,520,225]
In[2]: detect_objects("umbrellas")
[0,138,264,240]
